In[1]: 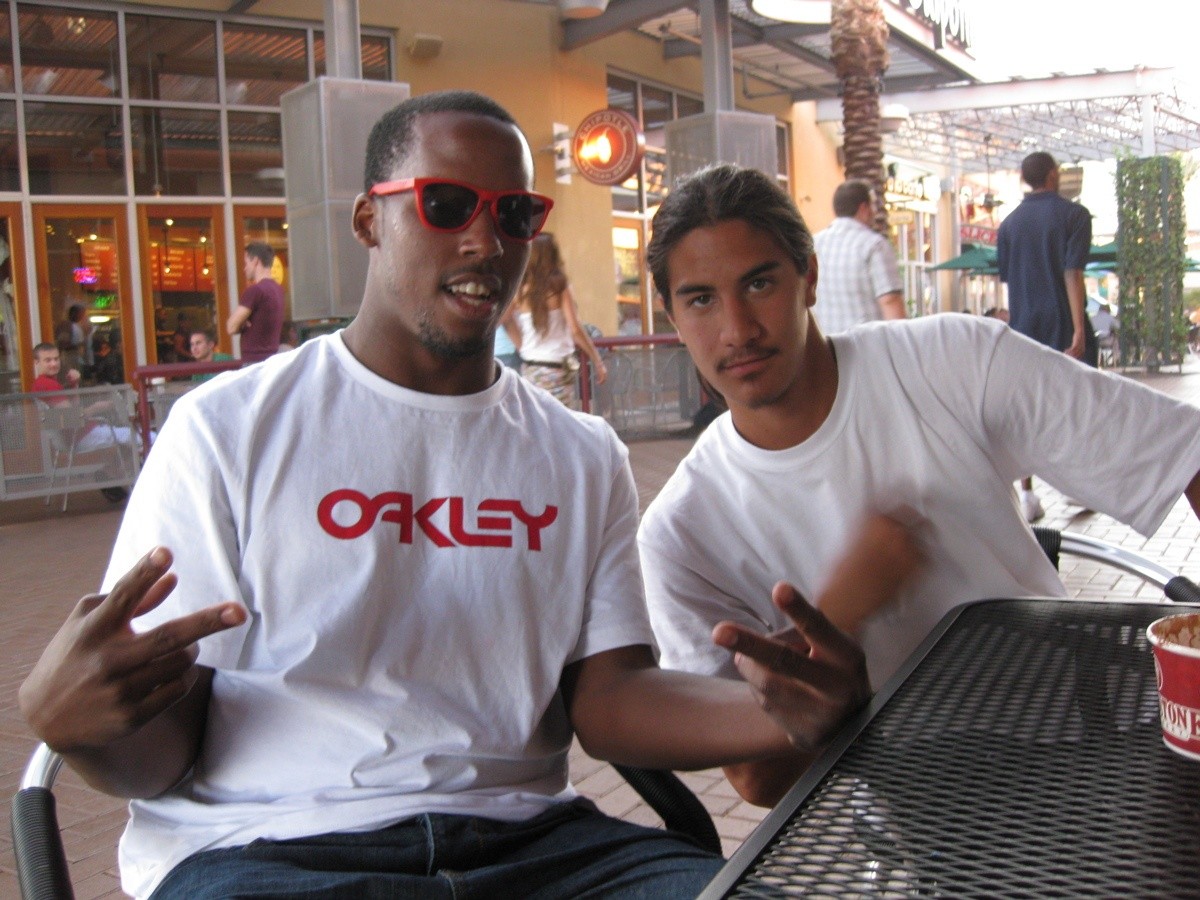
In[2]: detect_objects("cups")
[150,377,165,395]
[1146,610,1200,760]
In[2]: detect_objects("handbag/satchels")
[561,355,581,383]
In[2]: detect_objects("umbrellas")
[924,237,1199,280]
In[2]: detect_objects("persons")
[495,164,1199,900]
[810,181,907,338]
[227,240,286,371]
[997,151,1101,524]
[59,302,193,385]
[964,303,1200,368]
[17,88,876,900]
[31,324,297,501]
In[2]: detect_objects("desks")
[695,596,1200,900]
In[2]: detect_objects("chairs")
[610,347,701,435]
[10,741,723,900]
[32,381,204,512]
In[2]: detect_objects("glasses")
[368,176,554,245]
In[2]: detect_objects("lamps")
[406,33,444,59]
